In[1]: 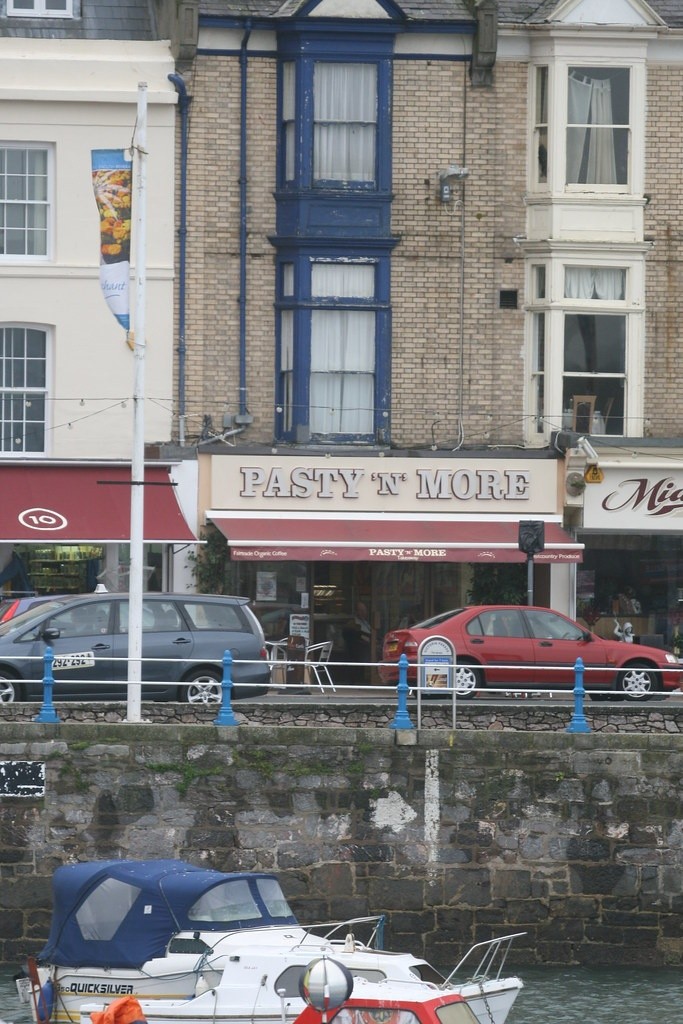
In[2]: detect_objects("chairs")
[572,393,598,434]
[264,635,337,694]
[602,395,616,425]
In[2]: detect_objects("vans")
[0,591,273,704]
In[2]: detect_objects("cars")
[0,594,70,630]
[379,603,683,701]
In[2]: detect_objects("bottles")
[194,975,210,998]
[37,980,54,1020]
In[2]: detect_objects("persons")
[614,621,635,643]
[344,601,376,672]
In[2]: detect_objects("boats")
[13,856,530,1024]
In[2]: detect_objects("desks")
[265,641,287,685]
[562,411,607,436]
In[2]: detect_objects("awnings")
[211,517,586,563]
[0,465,207,553]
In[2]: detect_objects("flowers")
[581,605,601,627]
